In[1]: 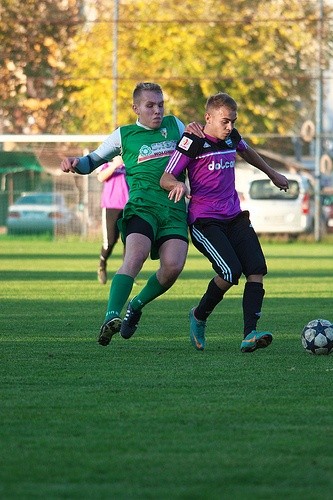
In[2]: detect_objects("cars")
[7,192,76,236]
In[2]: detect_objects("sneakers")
[120,296,142,339]
[97,317,123,346]
[189,306,207,351]
[240,329,273,353]
[98,263,106,284]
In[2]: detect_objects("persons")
[61,82,206,346]
[159,93,290,353]
[96,155,129,284]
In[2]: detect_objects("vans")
[240,175,315,239]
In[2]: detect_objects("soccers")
[300,318,333,356]
